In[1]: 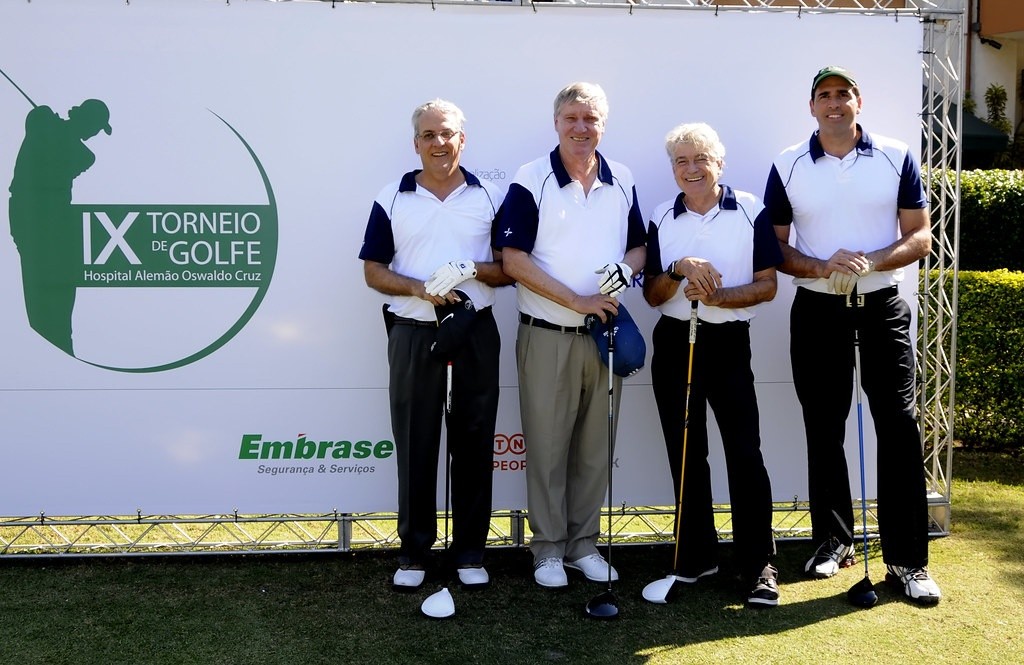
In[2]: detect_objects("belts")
[519,312,590,336]
[797,286,897,307]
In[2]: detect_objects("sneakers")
[393,558,426,590]
[805,536,857,577]
[454,562,489,586]
[534,556,569,586]
[666,562,719,582]
[748,564,779,606]
[887,564,939,602]
[564,551,619,582]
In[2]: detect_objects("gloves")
[827,256,871,296]
[595,263,633,297]
[424,260,476,296]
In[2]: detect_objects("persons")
[359,100,519,585]
[643,122,780,605]
[495,82,646,587]
[765,66,941,607]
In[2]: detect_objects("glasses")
[417,131,459,143]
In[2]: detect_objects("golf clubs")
[640,298,699,603]
[420,300,457,620]
[846,280,878,608]
[583,312,622,617]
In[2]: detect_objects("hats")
[433,288,478,351]
[585,300,646,379]
[812,66,857,89]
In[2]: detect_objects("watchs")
[667,260,685,281]
